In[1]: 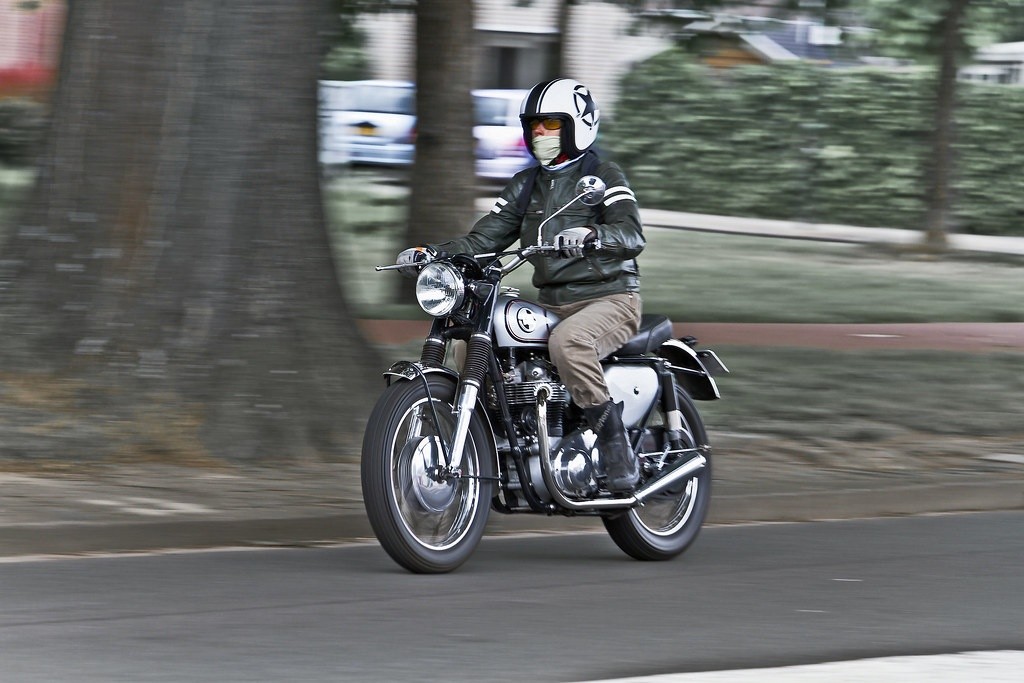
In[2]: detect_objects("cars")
[471,90,540,183]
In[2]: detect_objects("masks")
[532,136,564,165]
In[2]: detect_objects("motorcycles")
[359,175,731,574]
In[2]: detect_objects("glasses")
[529,119,566,130]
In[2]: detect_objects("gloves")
[395,246,433,278]
[553,226,596,260]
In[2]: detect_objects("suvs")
[318,77,416,168]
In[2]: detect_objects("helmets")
[520,78,602,158]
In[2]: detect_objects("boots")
[584,400,642,492]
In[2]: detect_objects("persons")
[396,79,648,494]
